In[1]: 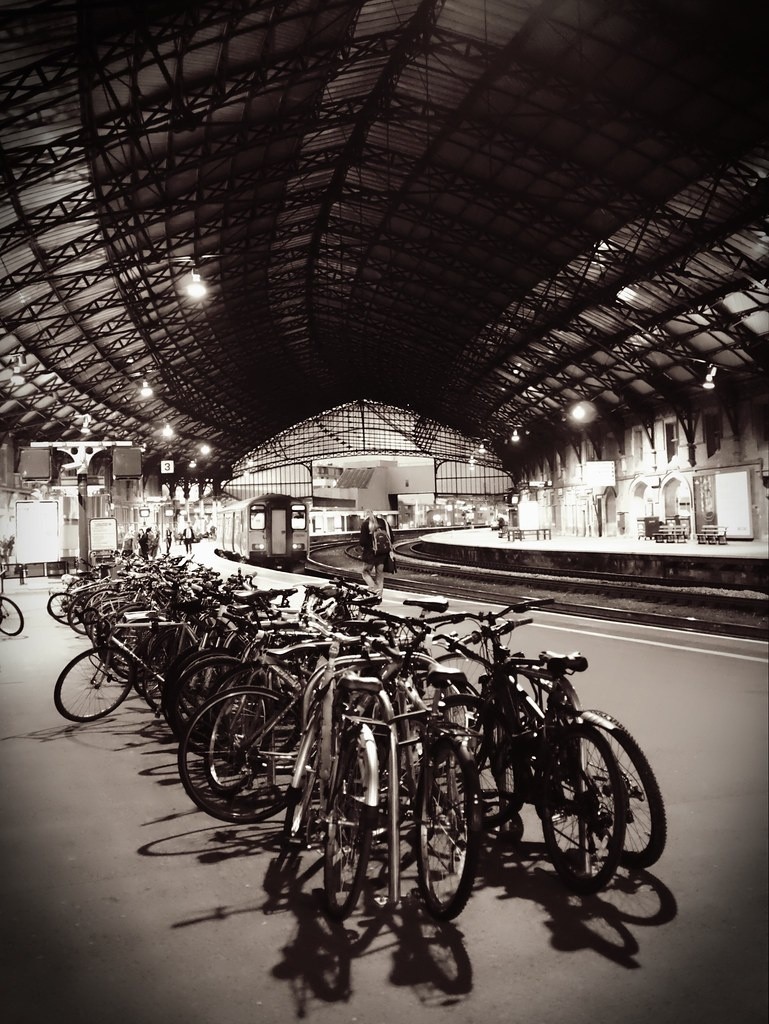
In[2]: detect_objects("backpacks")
[373,528,391,556]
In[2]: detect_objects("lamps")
[10,366,24,385]
[80,416,90,433]
[187,268,207,301]
[701,366,717,389]
[141,377,153,399]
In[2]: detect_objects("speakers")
[19,447,53,482]
[112,447,144,480]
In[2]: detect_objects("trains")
[216,493,309,571]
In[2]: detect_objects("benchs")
[652,525,688,543]
[508,529,551,542]
[697,525,728,545]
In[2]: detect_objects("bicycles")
[0,569,24,636]
[47,550,666,921]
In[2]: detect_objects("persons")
[121,525,151,560]
[183,522,195,554]
[497,515,505,529]
[164,525,173,553]
[0,534,15,577]
[358,508,394,605]
